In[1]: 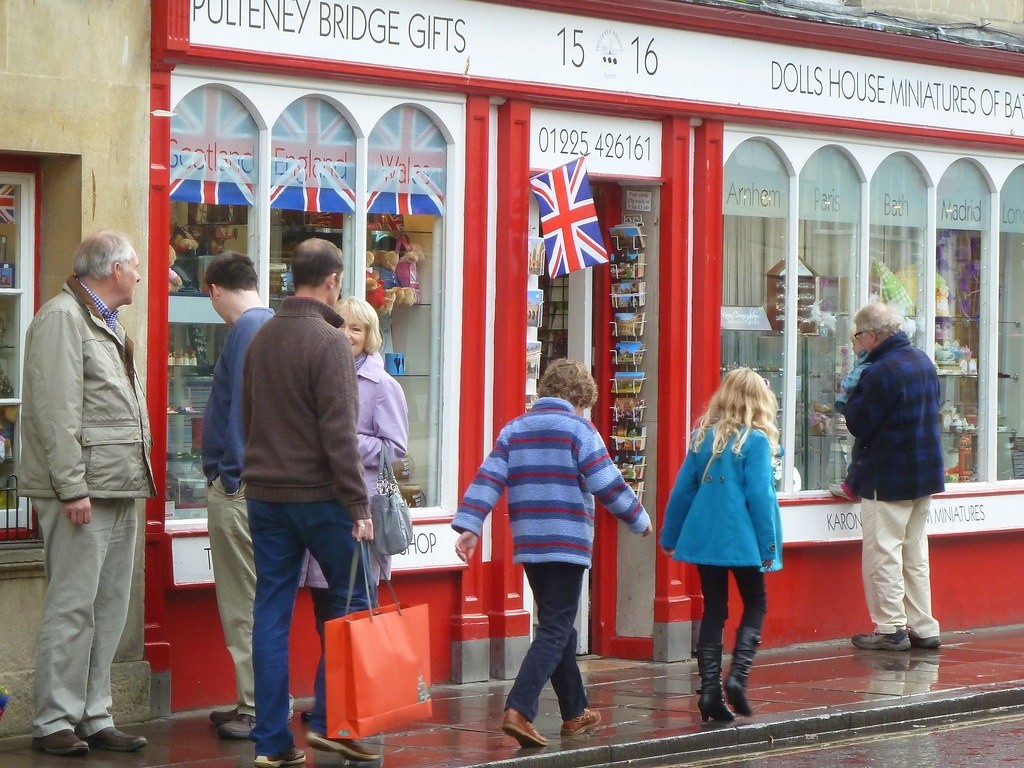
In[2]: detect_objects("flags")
[170,87,447,216]
[530,156,609,279]
[0,184,15,225]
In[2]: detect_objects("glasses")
[854,330,875,340]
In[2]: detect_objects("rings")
[359,524,366,528]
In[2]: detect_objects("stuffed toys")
[168,223,199,293]
[364,233,426,315]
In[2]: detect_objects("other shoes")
[210,706,294,739]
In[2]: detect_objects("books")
[525,236,546,411]
[608,225,646,504]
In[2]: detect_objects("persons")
[450,358,653,747]
[198,251,292,739]
[826,303,946,650]
[243,236,380,768]
[658,364,783,720]
[15,230,157,755]
[298,298,409,720]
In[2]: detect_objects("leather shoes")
[33,730,89,756]
[501,707,548,749]
[81,727,148,752]
[560,708,602,736]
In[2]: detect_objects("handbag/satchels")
[367,439,414,557]
[323,536,433,740]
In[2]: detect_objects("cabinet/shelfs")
[807,224,1024,490]
[167,199,443,523]
[0,155,41,540]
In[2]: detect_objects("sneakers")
[304,730,381,761]
[828,483,855,501]
[851,626,912,651]
[907,630,941,649]
[254,747,306,768]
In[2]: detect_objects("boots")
[725,625,763,717]
[696,642,737,722]
